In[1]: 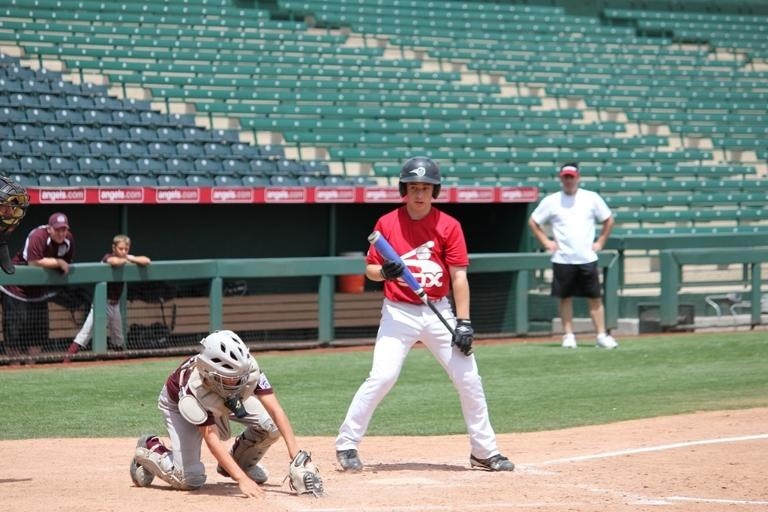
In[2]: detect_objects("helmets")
[0,174,30,275]
[399,157,440,200]
[197,329,252,403]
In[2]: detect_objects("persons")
[528,163,619,349]
[2,212,151,364]
[128,328,302,500]
[334,155,516,473]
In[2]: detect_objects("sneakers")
[217,450,268,485]
[595,335,619,349]
[336,449,363,474]
[562,333,577,349]
[469,454,514,471]
[130,433,156,487]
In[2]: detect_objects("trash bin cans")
[339,251,365,295]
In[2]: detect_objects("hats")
[559,166,579,177]
[49,213,70,230]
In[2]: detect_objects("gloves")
[380,259,404,281]
[451,319,474,355]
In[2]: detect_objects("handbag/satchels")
[126,322,176,350]
